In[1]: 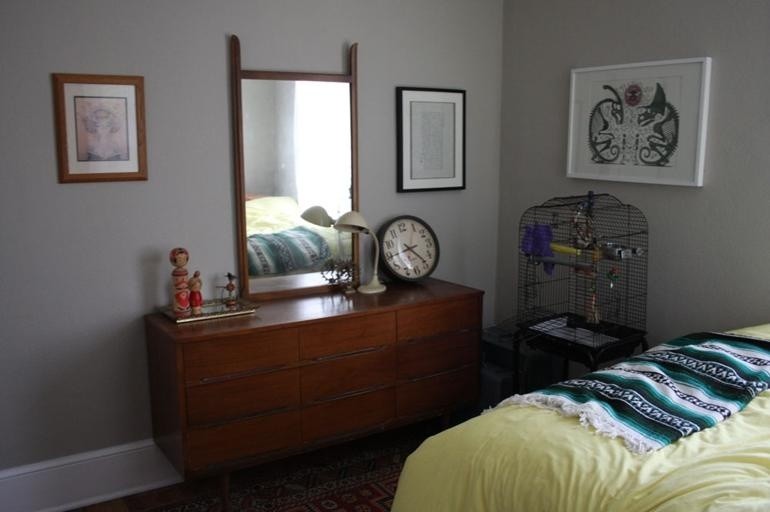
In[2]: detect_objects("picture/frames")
[565,57,711,187]
[396,87,467,194]
[51,73,147,184]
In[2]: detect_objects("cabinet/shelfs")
[142,277,485,495]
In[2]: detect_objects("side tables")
[510,313,647,393]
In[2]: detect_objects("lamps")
[334,212,386,294]
[300,206,347,262]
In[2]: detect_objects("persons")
[188,269,204,316]
[170,246,191,318]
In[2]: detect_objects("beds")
[391,323,770,511]
[244,195,354,280]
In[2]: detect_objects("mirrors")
[229,35,360,302]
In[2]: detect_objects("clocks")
[371,215,440,286]
